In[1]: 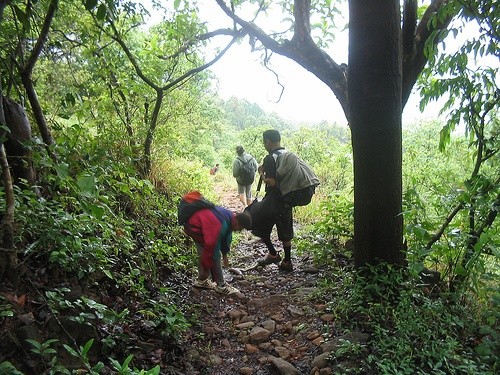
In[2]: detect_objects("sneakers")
[277,262,293,271]
[214,282,240,295]
[256,254,281,264]
[194,278,214,288]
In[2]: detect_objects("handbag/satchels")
[244,199,273,230]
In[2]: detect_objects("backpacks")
[271,150,315,205]
[178,191,230,236]
[236,157,255,185]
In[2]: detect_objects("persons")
[184,206,253,295]
[209,163,220,175]
[232,145,258,209]
[256,128,297,273]
